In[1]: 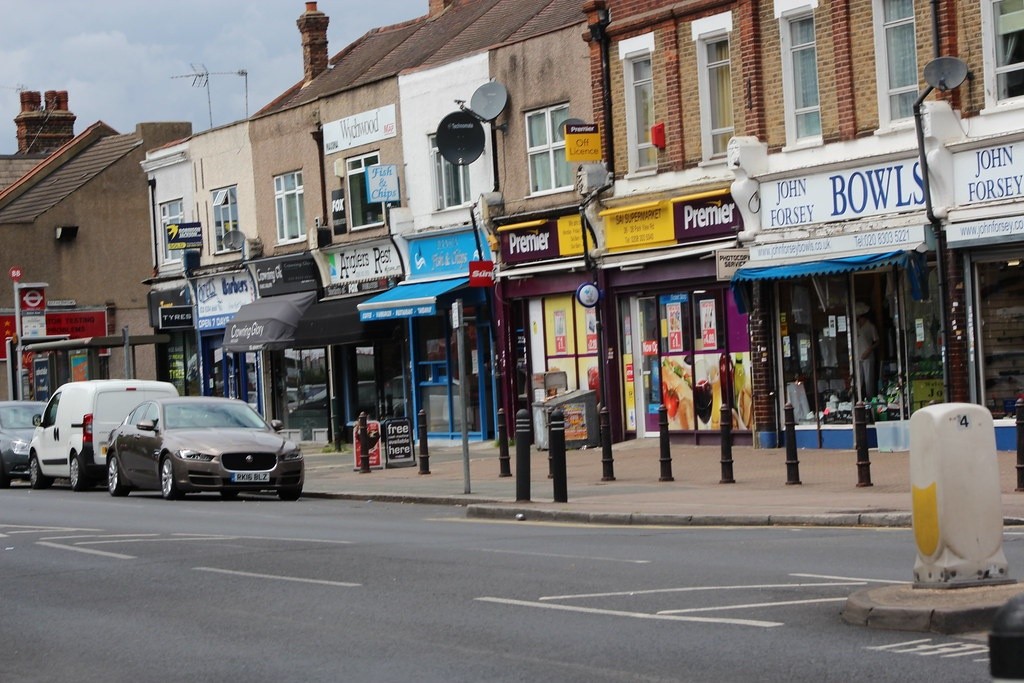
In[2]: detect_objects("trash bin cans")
[532,401,549,450]
[548,388,600,450]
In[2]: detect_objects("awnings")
[731,241,929,314]
[222,275,485,352]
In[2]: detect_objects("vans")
[27,376,181,492]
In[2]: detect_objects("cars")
[0,400,52,491]
[106,395,305,503]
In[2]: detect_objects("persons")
[483,357,498,439]
[852,304,880,403]
